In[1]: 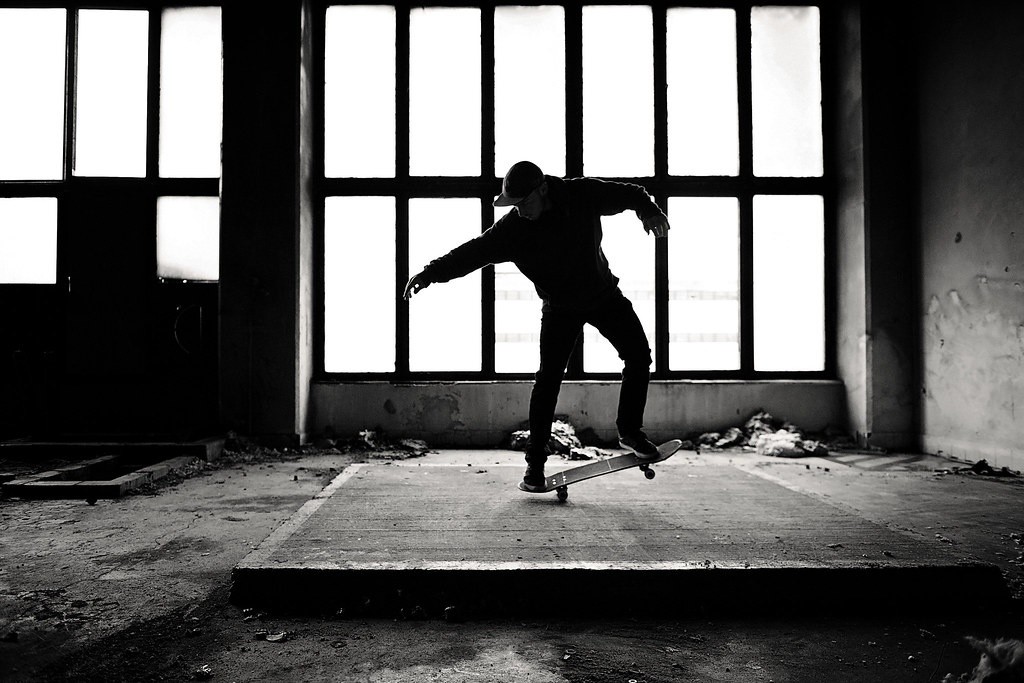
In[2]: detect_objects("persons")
[401,161,671,494]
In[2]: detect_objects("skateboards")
[518,439,683,500]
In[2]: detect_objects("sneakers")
[524,464,548,491]
[619,431,657,458]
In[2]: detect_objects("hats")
[493,161,545,206]
